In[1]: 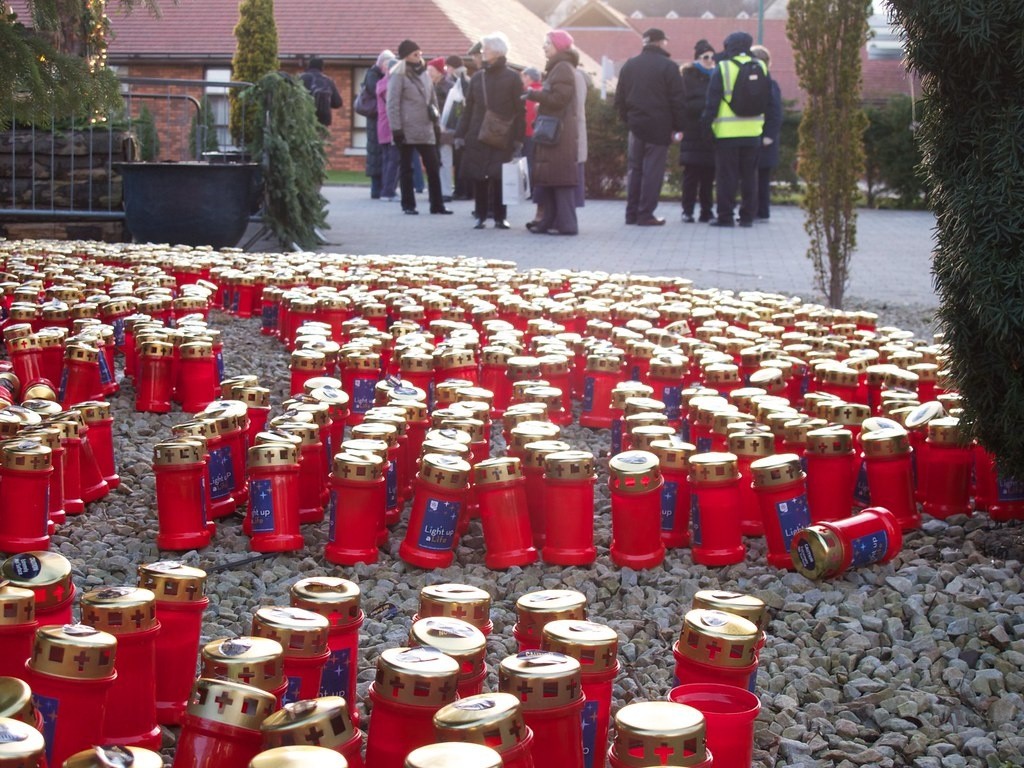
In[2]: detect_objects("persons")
[359,49,424,201]
[520,29,587,236]
[613,28,685,226]
[699,32,773,227]
[387,39,453,215]
[520,66,543,156]
[454,31,526,230]
[301,58,343,127]
[751,46,781,219]
[426,55,471,202]
[679,39,716,223]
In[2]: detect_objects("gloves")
[700,122,711,129]
[521,87,532,101]
[511,141,523,158]
[434,125,440,141]
[393,130,404,144]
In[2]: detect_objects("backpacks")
[722,58,768,115]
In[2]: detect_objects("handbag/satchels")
[428,104,439,120]
[313,90,331,125]
[440,78,466,133]
[531,114,563,146]
[478,110,512,148]
[353,89,377,119]
[502,154,531,206]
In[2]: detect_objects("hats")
[310,59,322,70]
[548,31,573,52]
[694,40,715,59]
[643,29,670,44]
[399,39,482,68]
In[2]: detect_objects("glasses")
[700,55,714,60]
[480,46,492,53]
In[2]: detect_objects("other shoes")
[474,214,576,236]
[372,193,472,215]
[626,208,769,226]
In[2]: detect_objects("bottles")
[0,231,1020,768]
